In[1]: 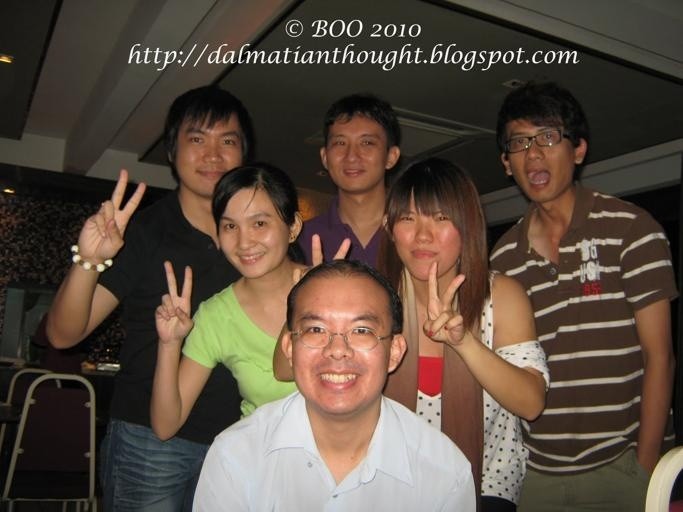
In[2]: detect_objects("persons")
[150,163,313,442]
[487,84,678,512]
[273,157,551,512]
[45,86,255,512]
[193,259,476,512]
[296,94,401,279]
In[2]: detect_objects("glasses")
[291,324,392,351]
[505,128,574,157]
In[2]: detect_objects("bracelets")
[70,243,113,273]
